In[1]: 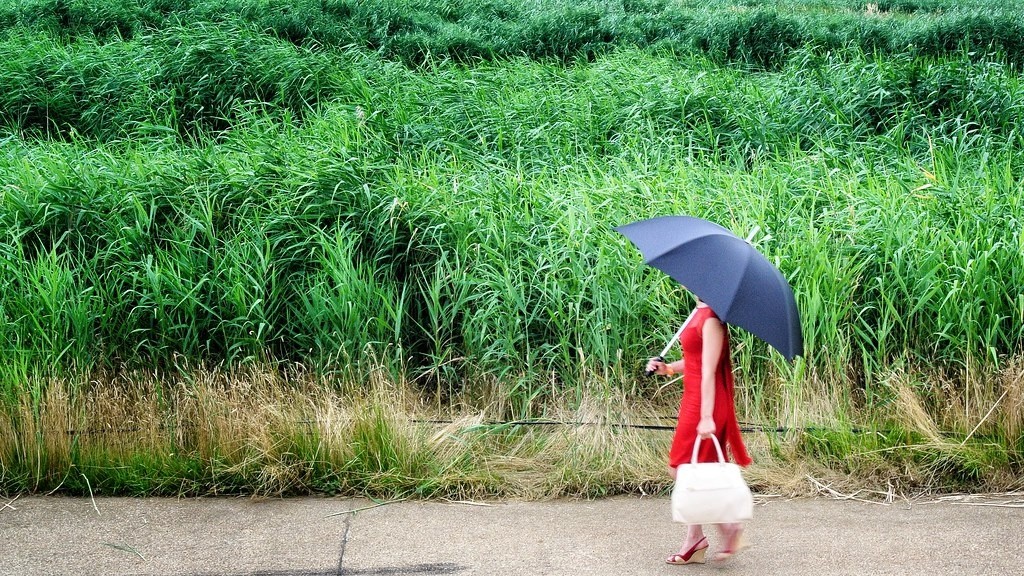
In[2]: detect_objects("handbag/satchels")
[670,433,753,525]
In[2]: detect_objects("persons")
[645,274,752,564]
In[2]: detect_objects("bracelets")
[666,363,674,377]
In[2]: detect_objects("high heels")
[715,524,745,562]
[666,537,709,564]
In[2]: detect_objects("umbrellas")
[616,216,805,377]
